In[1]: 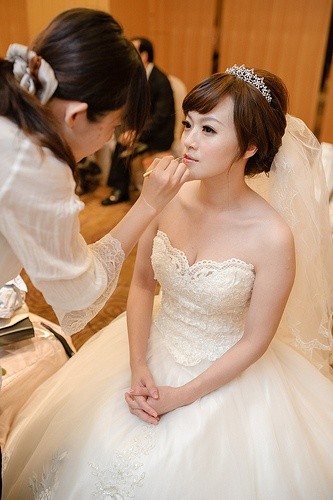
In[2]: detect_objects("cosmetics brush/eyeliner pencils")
[143,154,187,178]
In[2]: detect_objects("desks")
[0,312,76,450]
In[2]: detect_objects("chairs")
[132,75,188,188]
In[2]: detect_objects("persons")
[0,7,190,337]
[101,37,175,206]
[0,64,332,499]
